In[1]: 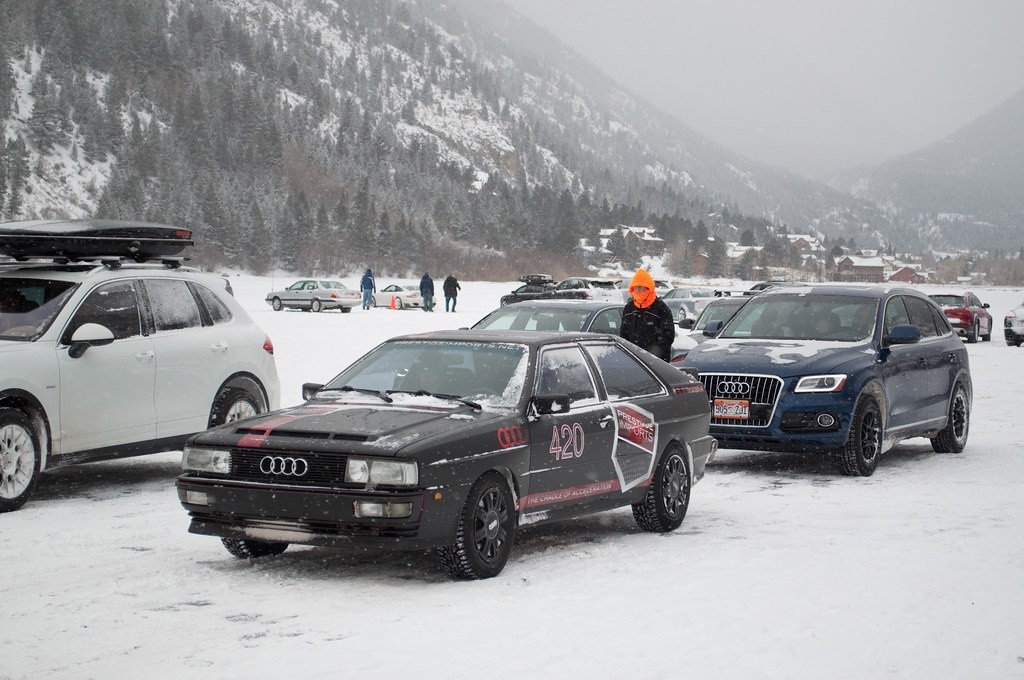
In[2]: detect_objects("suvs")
[682,284,974,478]
[543,276,625,300]
[0,219,283,511]
[500,274,558,305]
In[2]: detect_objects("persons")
[619,268,676,365]
[442,274,461,312]
[360,269,376,310]
[420,273,434,312]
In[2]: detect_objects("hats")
[629,270,655,308]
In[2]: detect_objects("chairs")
[536,317,560,331]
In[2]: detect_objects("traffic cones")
[389,297,397,310]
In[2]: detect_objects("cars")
[926,291,993,343]
[621,274,793,343]
[458,299,698,363]
[172,328,718,581]
[265,277,364,313]
[371,283,438,308]
[1003,301,1024,347]
